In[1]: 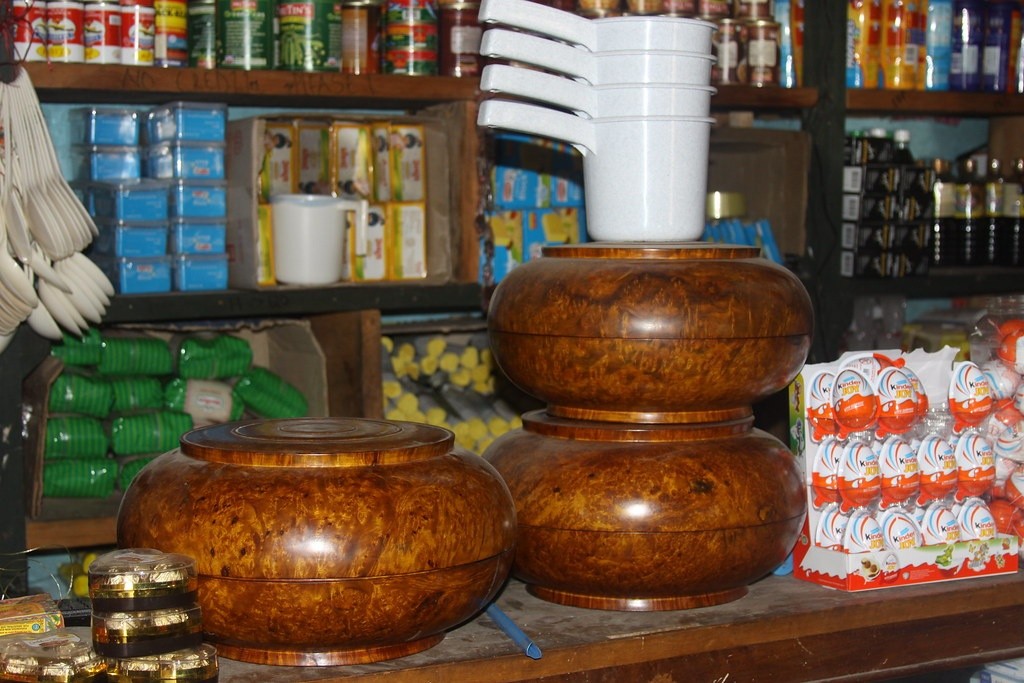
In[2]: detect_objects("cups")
[267,192,369,285]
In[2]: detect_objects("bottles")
[848,127,1024,266]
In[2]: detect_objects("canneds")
[540,0,807,90]
[843,0,1013,94]
[13,0,487,79]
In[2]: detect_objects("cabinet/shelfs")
[0,61,1024,552]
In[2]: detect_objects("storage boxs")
[20,317,330,522]
[217,106,453,293]
[708,126,808,255]
[779,347,1018,591]
